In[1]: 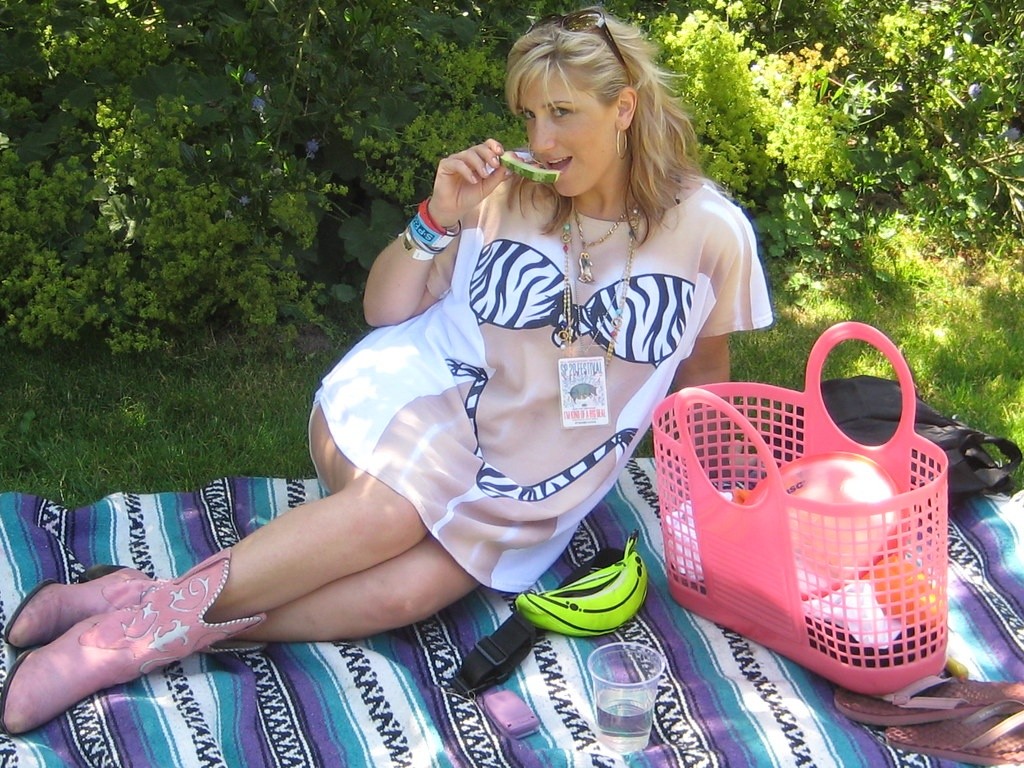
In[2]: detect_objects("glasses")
[526,9,633,86]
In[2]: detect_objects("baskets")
[651,321,949,695]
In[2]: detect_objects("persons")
[0,8,774,736]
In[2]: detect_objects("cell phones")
[485,689,540,739]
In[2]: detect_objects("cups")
[588,642,666,756]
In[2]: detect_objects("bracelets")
[403,197,461,261]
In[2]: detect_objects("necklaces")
[559,203,642,372]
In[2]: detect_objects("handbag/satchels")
[761,375,1022,498]
[447,528,648,700]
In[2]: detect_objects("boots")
[4,563,266,653]
[0,547,268,738]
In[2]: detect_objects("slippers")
[833,675,1024,727]
[884,700,1024,765]
